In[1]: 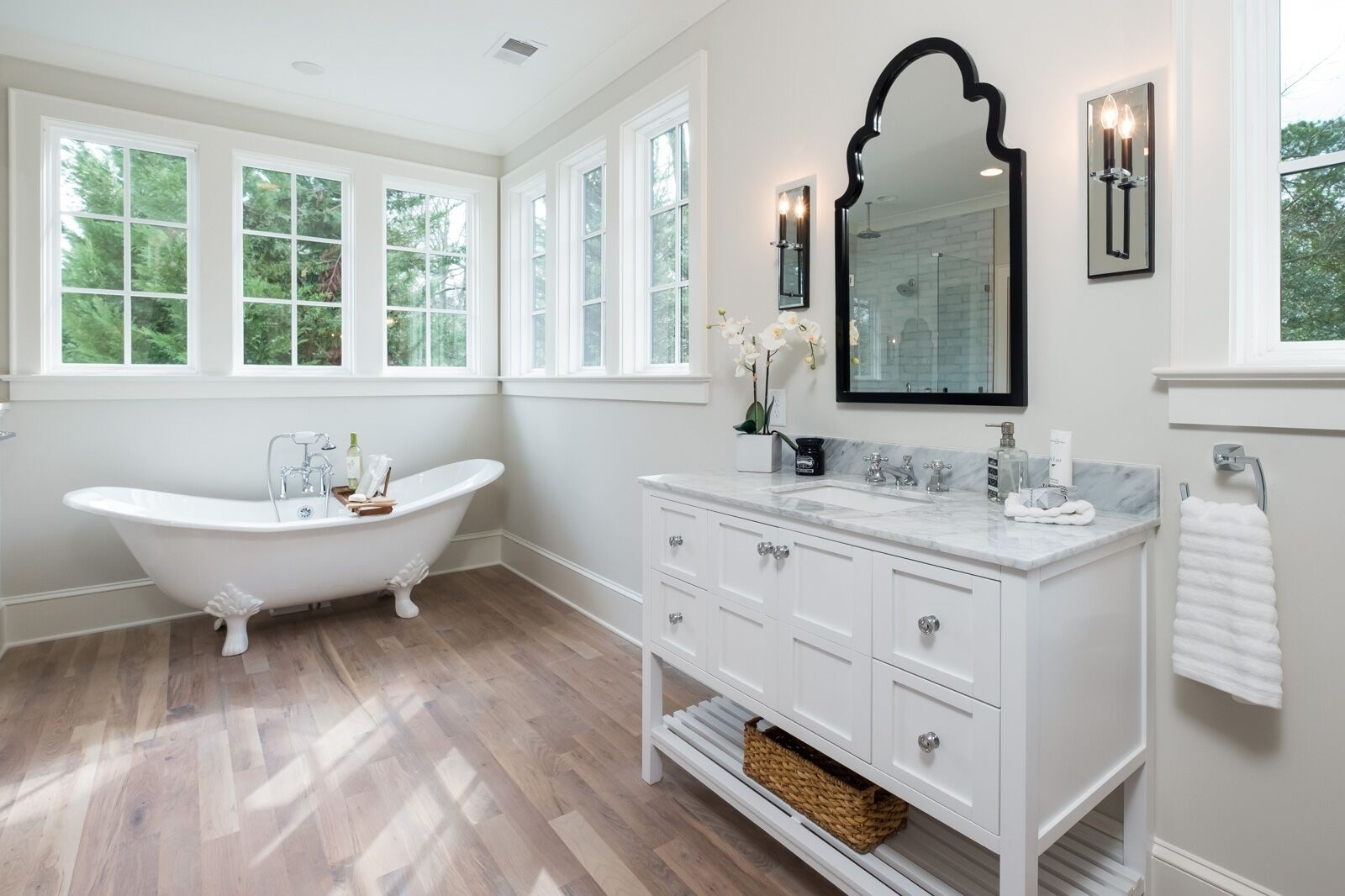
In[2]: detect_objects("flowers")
[705,307,828,451]
[850,319,860,367]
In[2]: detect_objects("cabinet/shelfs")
[633,462,1151,896]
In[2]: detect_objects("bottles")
[346,432,363,491]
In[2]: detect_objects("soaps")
[348,494,366,502]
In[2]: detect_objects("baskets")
[743,716,911,852]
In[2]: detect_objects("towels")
[1170,495,1284,710]
[1005,490,1096,526]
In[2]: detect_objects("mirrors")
[1086,81,1154,281]
[835,36,1027,410]
[897,315,932,383]
[931,250,989,393]
[775,184,810,310]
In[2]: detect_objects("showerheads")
[283,432,336,447]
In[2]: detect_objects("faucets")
[297,466,315,494]
[876,454,918,488]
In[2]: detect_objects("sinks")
[769,479,951,516]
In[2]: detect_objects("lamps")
[857,202,881,240]
[1111,103,1144,259]
[1093,93,1131,258]
[770,192,795,297]
[790,195,803,297]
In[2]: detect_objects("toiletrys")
[347,432,362,491]
[985,421,1031,504]
[1047,429,1074,486]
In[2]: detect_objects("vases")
[733,431,782,473]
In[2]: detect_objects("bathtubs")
[62,457,505,657]
